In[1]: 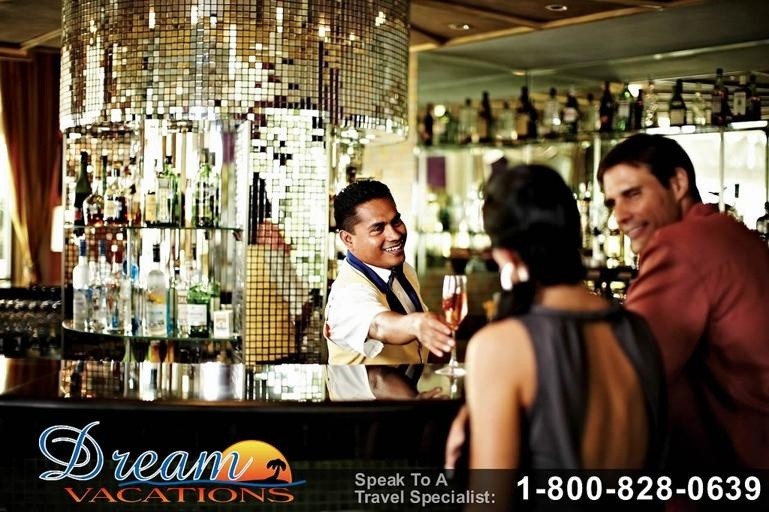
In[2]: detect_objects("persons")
[595,132,767,507]
[246,172,311,365]
[441,162,679,510]
[320,177,457,367]
[322,360,452,401]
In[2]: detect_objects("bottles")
[421,69,762,146]
[69,145,250,407]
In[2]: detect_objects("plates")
[408,1,769,284]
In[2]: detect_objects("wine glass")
[435,275,473,378]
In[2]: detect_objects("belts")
[435,275,473,378]
